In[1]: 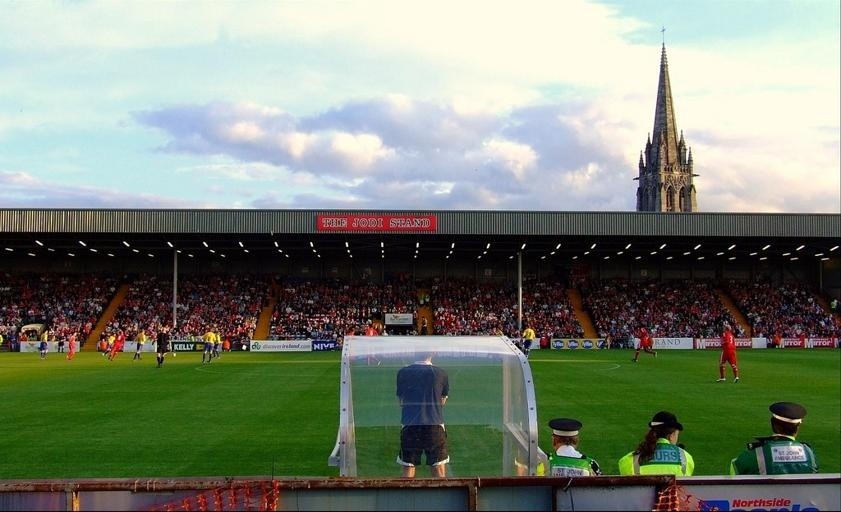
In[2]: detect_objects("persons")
[800,329,816,348]
[731,400,821,475]
[38,330,48,359]
[213,331,221,359]
[100,329,125,361]
[201,328,216,363]
[522,325,535,359]
[773,331,784,348]
[721,277,840,337]
[577,278,747,350]
[431,278,584,339]
[632,323,657,362]
[132,329,146,360]
[619,411,696,477]
[395,349,457,478]
[66,333,76,360]
[715,321,740,382]
[2,271,121,353]
[829,333,840,348]
[99,273,272,352]
[271,276,430,339]
[156,328,170,367]
[538,418,604,477]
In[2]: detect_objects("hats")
[769,401,806,424]
[647,411,683,432]
[549,418,582,437]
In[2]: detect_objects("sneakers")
[715,378,727,382]
[734,377,740,383]
